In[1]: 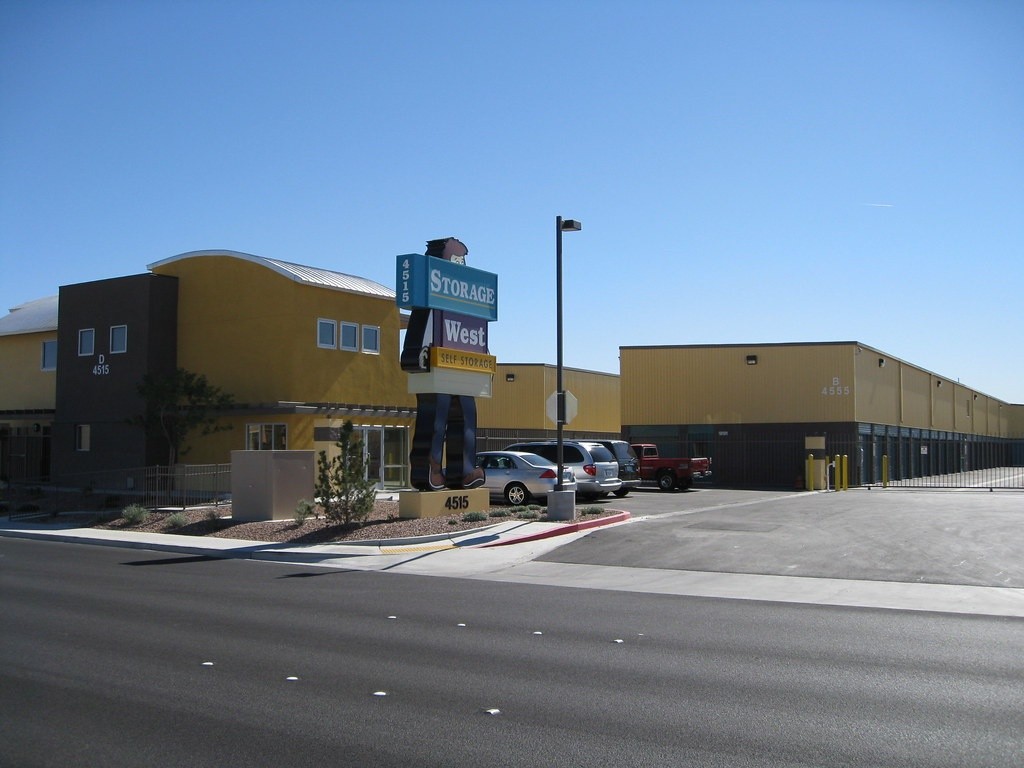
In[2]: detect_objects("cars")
[440,450,576,506]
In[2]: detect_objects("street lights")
[549,214,581,522]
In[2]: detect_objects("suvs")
[505,442,622,503]
[575,437,640,498]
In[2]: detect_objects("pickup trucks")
[633,442,712,492]
